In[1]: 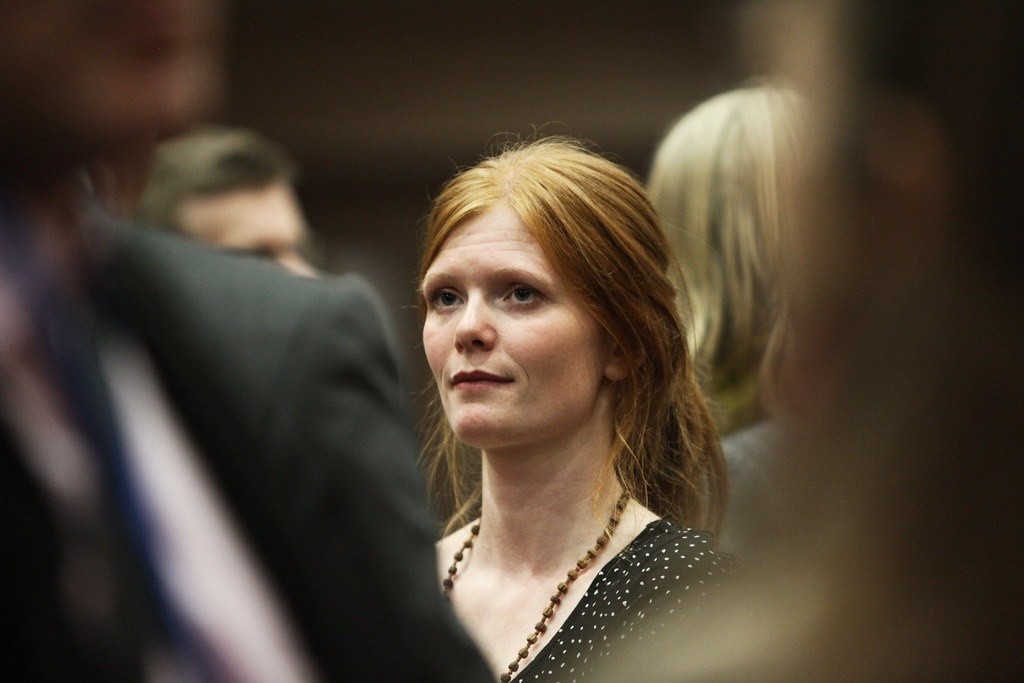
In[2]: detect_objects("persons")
[573,1,1023,683]
[640,79,832,557]
[0,1,499,681]
[416,140,765,683]
[142,117,368,295]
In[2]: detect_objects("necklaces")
[441,486,634,683]
